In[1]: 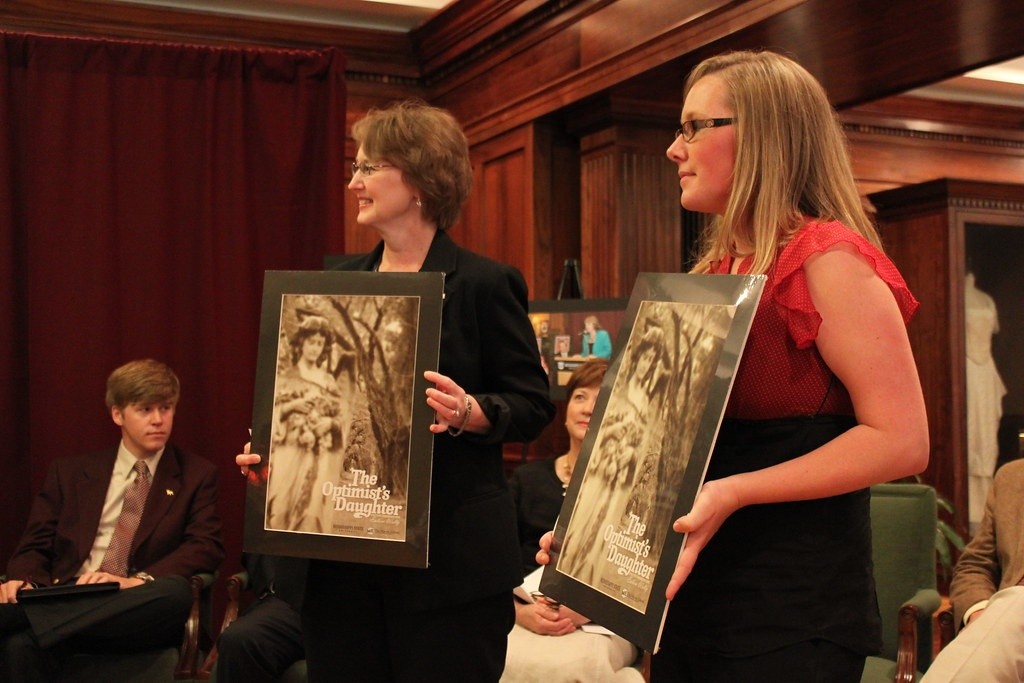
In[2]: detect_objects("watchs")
[136,572,154,581]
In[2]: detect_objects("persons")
[235,102,552,683]
[1,359,224,682]
[965,271,1006,535]
[216,556,304,683]
[265,318,347,532]
[498,52,929,682]
[555,340,575,370]
[921,457,1024,683]
[574,316,612,359]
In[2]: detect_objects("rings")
[454,410,459,417]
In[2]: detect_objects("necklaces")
[561,464,569,496]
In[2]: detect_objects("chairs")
[170,571,219,683]
[196,568,307,683]
[858,481,939,683]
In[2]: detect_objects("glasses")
[674,117,738,143]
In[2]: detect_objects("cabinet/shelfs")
[866,176,1024,551]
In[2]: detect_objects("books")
[513,565,544,604]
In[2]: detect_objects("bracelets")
[447,397,472,437]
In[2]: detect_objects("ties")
[97,460,152,578]
